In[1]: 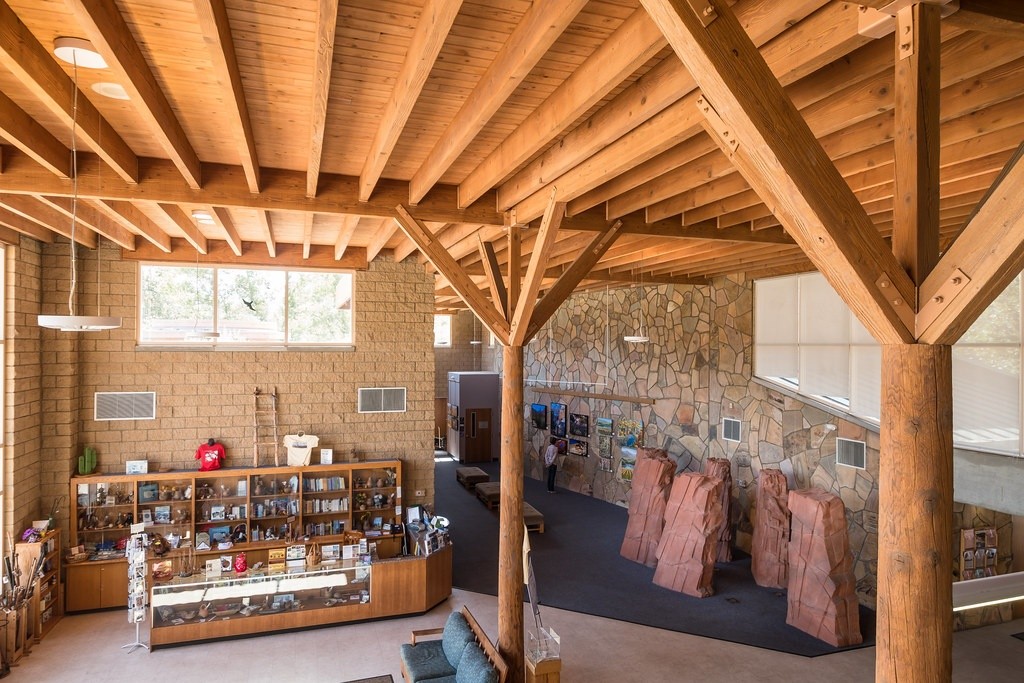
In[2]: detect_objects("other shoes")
[547,491,550,492]
[550,491,556,493]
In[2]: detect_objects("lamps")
[624,283,650,344]
[37,35,121,332]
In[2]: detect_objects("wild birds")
[242,299,256,311]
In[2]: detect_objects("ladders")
[252,386,279,468]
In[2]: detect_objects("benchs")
[399,605,509,683]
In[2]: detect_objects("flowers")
[22,528,39,543]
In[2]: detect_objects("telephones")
[390,523,402,534]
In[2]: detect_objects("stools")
[524,502,544,533]
[455,466,488,489]
[475,482,500,509]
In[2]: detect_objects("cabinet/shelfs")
[0,525,65,666]
[59,460,451,655]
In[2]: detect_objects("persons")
[544,437,560,494]
